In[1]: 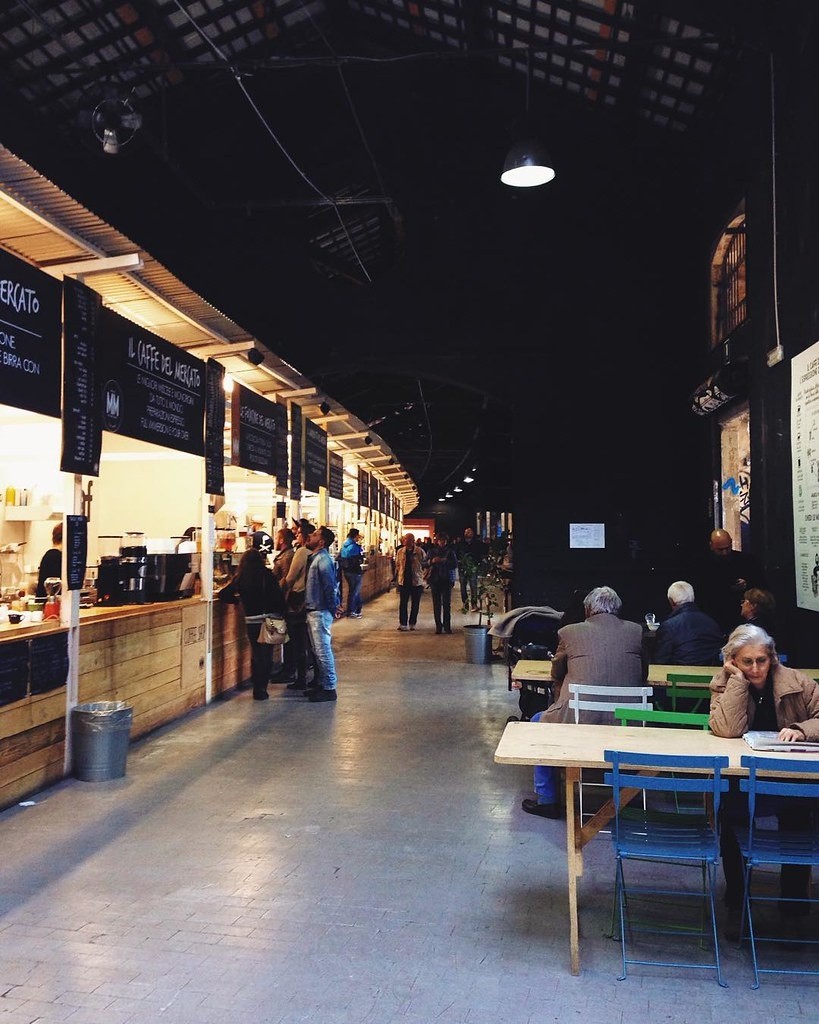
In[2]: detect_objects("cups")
[31,603,43,622]
[8,614,26,624]
[644,613,656,623]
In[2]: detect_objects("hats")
[292,517,308,527]
[252,516,264,523]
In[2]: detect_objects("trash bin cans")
[69,700,135,783]
[462,624,492,665]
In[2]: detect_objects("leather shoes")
[626,799,645,809]
[522,799,563,819]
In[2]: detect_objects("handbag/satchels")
[426,564,442,583]
[258,618,290,645]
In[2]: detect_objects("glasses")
[740,600,748,604]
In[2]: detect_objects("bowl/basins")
[647,623,661,631]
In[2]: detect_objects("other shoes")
[461,603,470,614]
[470,606,478,613]
[396,624,416,631]
[253,689,269,701]
[308,678,322,687]
[287,679,306,690]
[780,915,806,950]
[436,626,452,634]
[346,611,363,618]
[725,907,751,940]
[308,665,314,670]
[271,673,296,683]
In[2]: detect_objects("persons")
[708,624,819,951]
[391,536,494,593]
[456,527,482,614]
[394,532,458,635]
[218,518,343,703]
[504,529,792,820]
[335,528,364,619]
[34,522,63,604]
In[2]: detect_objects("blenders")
[43,577,61,619]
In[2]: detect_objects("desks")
[511,659,818,718]
[493,722,819,971]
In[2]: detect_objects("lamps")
[453,482,463,492]
[416,494,420,497]
[500,49,557,186]
[390,458,395,464]
[446,488,455,498]
[464,471,476,484]
[404,474,410,479]
[320,402,331,416]
[412,486,416,491]
[438,493,446,502]
[246,349,264,366]
[365,436,372,445]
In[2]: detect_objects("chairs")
[569,684,656,835]
[603,752,734,988]
[666,672,718,813]
[741,755,819,990]
[607,708,710,948]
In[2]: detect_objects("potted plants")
[459,548,506,663]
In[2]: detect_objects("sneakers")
[304,685,324,697]
[309,688,337,702]
